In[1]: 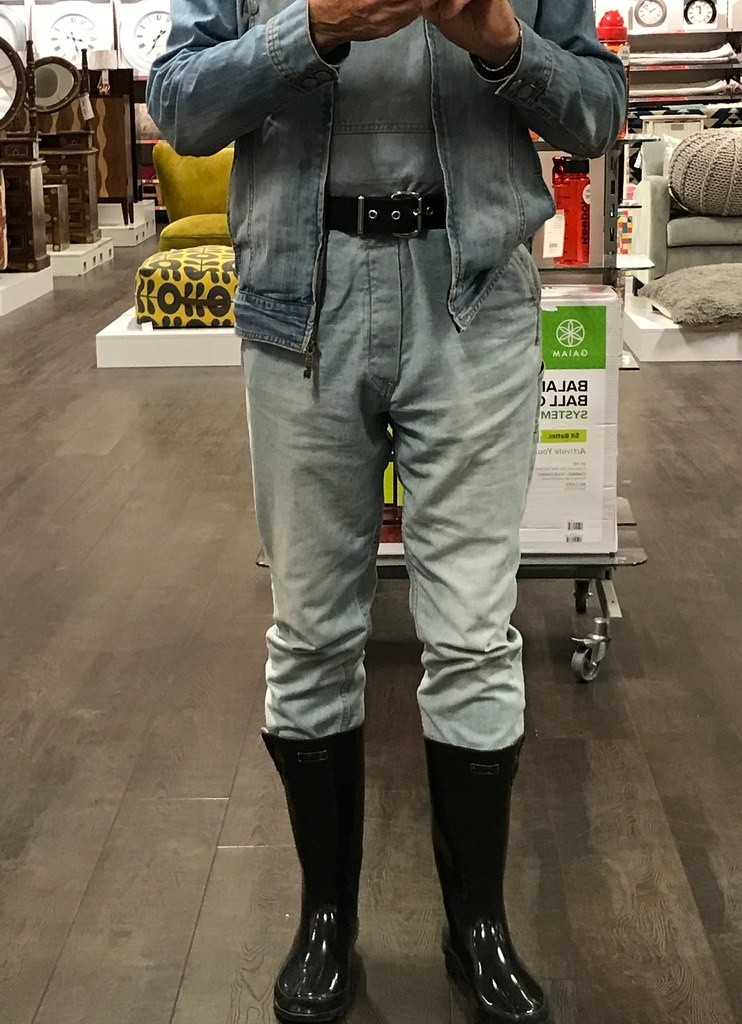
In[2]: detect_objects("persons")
[146,0,628,1024]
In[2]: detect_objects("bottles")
[596,10,630,140]
[552,155,591,268]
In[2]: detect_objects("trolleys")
[247,9,651,683]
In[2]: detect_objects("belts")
[325,190,446,239]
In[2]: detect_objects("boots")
[259,722,366,1023]
[423,734,551,1023]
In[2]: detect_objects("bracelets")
[477,40,520,78]
